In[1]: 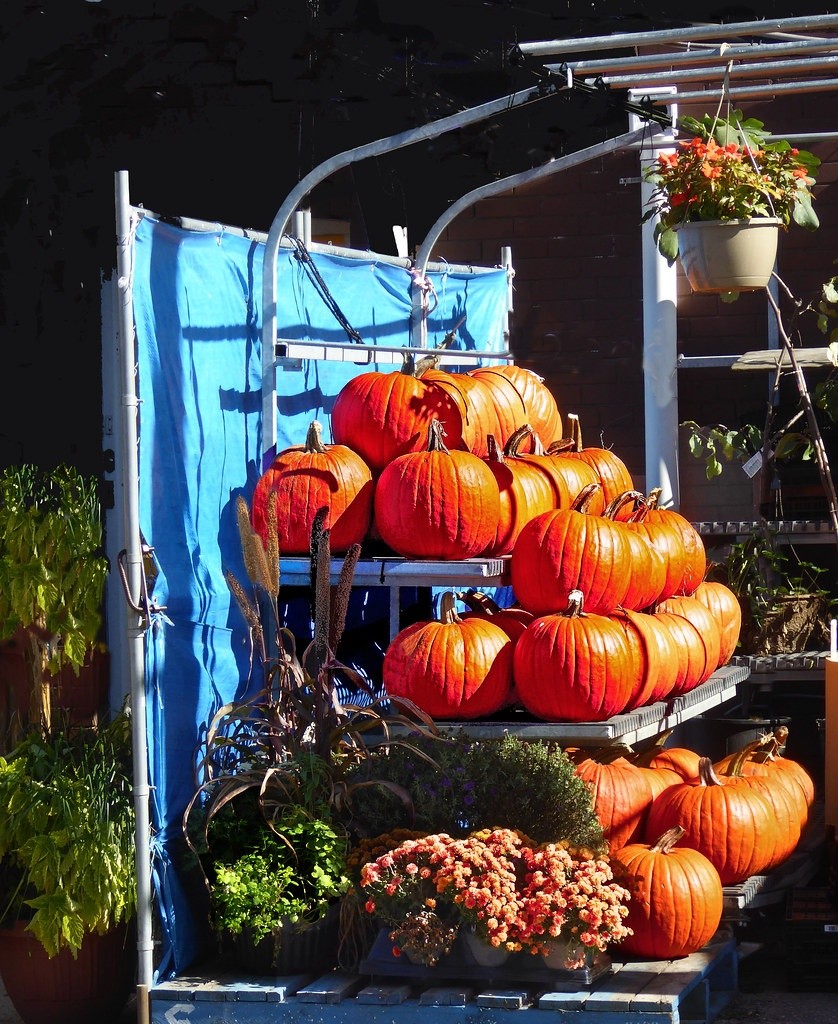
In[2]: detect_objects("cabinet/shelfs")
[104,13,838,1023]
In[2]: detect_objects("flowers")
[637,100,821,267]
[336,724,634,966]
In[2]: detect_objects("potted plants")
[0,456,141,1024]
[179,492,391,978]
[707,514,830,655]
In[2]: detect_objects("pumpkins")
[549,726,814,959]
[252,344,741,722]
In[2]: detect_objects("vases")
[1,641,110,752]
[670,217,783,293]
[686,712,791,765]
[393,921,603,971]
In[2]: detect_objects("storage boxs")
[784,887,838,993]
